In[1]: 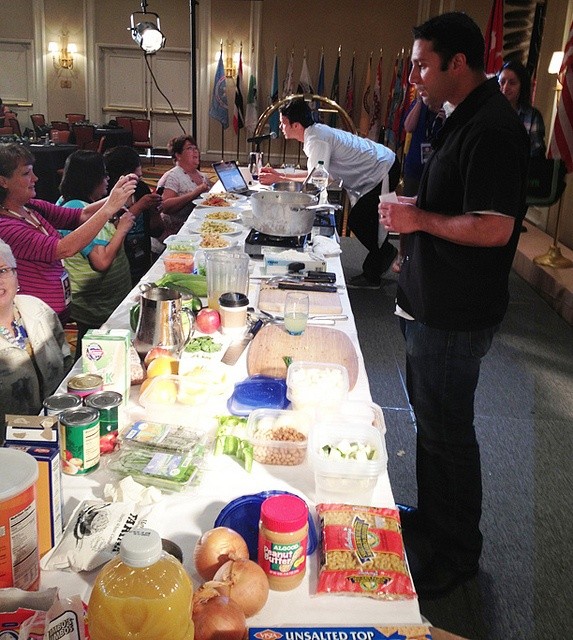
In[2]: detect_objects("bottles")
[80,328,132,407]
[81,526,196,635]
[310,159,330,204]
[219,292,250,336]
[1,446,40,591]
[256,492,309,592]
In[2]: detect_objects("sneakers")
[346,274,380,290]
[362,243,398,276]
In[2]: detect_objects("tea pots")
[206,250,249,306]
[131,286,195,355]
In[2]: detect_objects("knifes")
[219,319,263,366]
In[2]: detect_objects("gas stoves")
[245,230,312,259]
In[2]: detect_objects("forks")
[260,311,336,327]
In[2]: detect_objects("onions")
[194,526,251,582]
[203,558,270,619]
[193,589,249,640]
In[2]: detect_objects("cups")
[283,293,309,336]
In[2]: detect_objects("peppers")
[129,302,143,327]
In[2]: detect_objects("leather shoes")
[415,555,480,598]
[394,502,420,530]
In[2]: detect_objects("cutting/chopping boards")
[246,322,358,392]
[256,279,343,315]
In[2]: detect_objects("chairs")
[8,119,22,134]
[115,116,134,128]
[69,124,93,148]
[0,126,11,136]
[31,114,50,135]
[64,113,86,124]
[128,118,155,164]
[51,121,68,132]
[49,129,71,146]
[68,125,131,148]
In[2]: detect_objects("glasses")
[0,267,15,279]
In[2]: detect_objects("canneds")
[65,372,105,398]
[59,407,102,477]
[86,391,124,456]
[42,391,83,419]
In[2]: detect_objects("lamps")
[126,0,165,55]
[214,37,245,79]
[47,26,79,71]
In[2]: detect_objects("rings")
[121,186,127,190]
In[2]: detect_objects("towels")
[313,234,343,256]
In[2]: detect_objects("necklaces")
[2,204,48,236]
[0,320,21,342]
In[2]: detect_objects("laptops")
[211,161,259,195]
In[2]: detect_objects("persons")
[393,85,454,273]
[56,148,134,330]
[155,135,213,235]
[378,13,530,601]
[0,142,138,330]
[98,135,135,152]
[0,239,72,439]
[496,61,546,184]
[258,99,402,290]
[101,147,165,287]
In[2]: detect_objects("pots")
[272,183,321,204]
[242,190,341,236]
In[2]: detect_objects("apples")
[196,307,222,334]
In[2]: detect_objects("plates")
[200,192,248,200]
[186,220,243,234]
[190,207,241,219]
[192,199,240,207]
[193,235,239,249]
